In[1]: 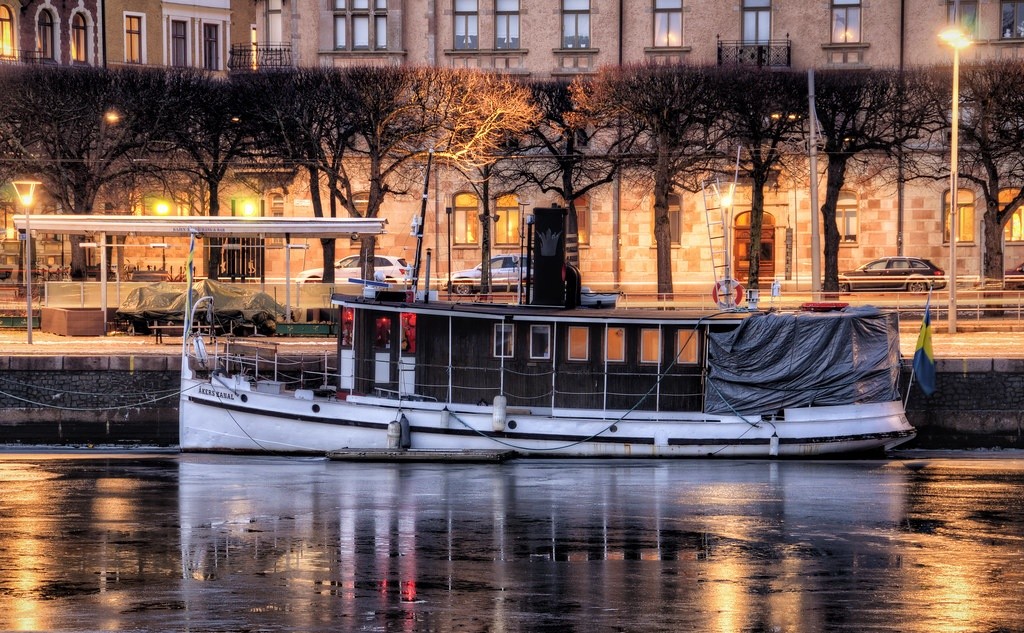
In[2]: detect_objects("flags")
[912,295,936,397]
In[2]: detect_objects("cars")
[1005,263,1024,290]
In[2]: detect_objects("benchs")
[147,325,221,345]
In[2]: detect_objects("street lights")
[940,27,973,339]
[10,180,42,344]
[156,203,169,271]
[720,195,734,302]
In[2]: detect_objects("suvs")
[441,253,533,295]
[295,255,414,284]
[819,256,947,295]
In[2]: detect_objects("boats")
[179,235,917,458]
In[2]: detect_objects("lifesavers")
[713,279,742,308]
